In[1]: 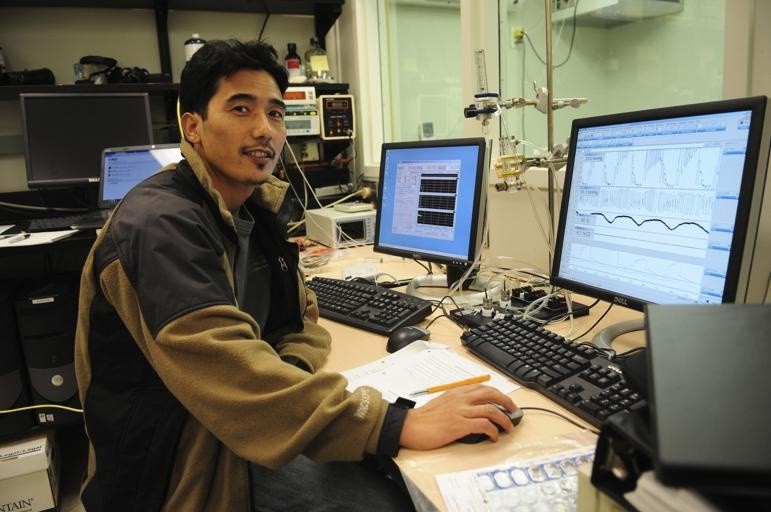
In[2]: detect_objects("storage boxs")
[0,428,64,512]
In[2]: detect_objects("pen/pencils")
[410,375,491,396]
[9,234,31,242]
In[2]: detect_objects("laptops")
[98,143,186,222]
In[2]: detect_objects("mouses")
[457,404,523,443]
[386,326,430,354]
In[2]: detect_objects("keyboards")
[28,216,102,231]
[461,312,648,429]
[304,276,433,336]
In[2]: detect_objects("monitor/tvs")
[19,92,154,187]
[373,136,503,306]
[550,95,767,365]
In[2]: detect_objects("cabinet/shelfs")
[0,0,355,206]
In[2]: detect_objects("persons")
[74,38,519,512]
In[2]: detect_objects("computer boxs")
[0,292,36,443]
[14,275,77,426]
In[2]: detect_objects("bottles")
[284,41,303,80]
[304,36,332,80]
[184,32,206,63]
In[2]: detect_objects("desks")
[294,240,649,512]
[0,209,98,278]
